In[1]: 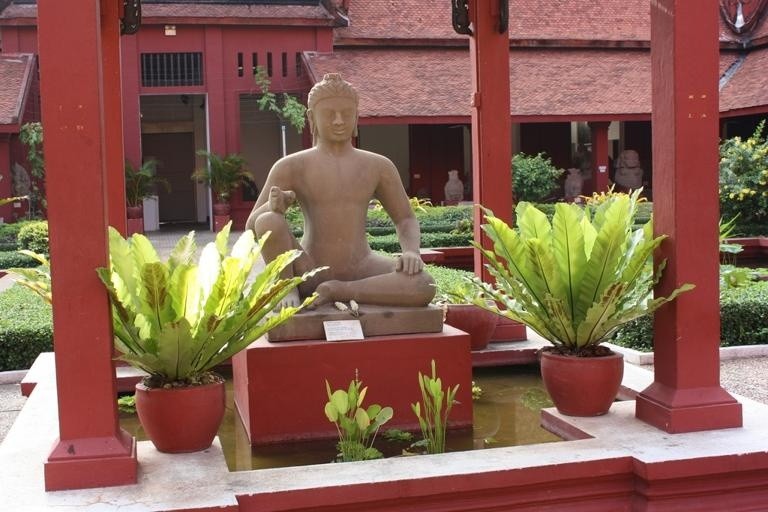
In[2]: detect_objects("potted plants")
[125,157,172,218]
[189,150,256,215]
[424,187,698,417]
[1,223,329,452]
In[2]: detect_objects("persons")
[245,73,436,311]
